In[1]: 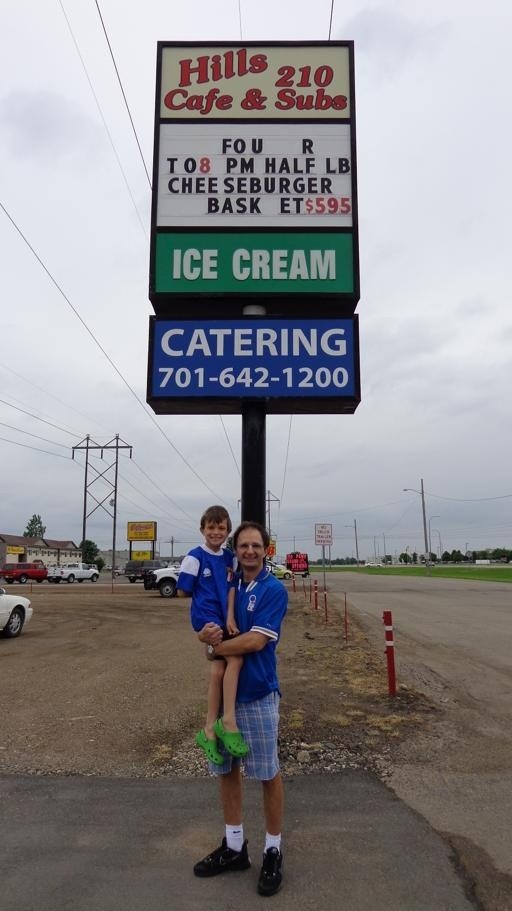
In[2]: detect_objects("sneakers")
[258,847,283,896]
[193,837,250,877]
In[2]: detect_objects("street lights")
[429,515,441,552]
[402,489,428,558]
[465,542,469,557]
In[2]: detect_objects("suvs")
[140,564,183,599]
[125,559,166,583]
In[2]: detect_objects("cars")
[421,560,439,567]
[103,559,181,577]
[364,561,383,569]
[266,560,294,579]
[0,585,34,640]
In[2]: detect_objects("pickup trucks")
[44,560,100,584]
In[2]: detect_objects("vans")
[3,560,49,584]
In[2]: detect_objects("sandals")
[195,719,249,764]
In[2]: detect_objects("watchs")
[208,645,214,658]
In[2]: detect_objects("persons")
[175,506,249,765]
[194,521,288,896]
